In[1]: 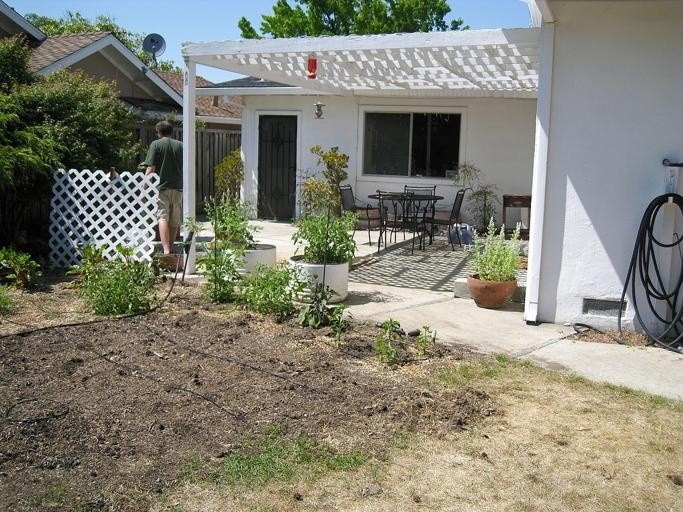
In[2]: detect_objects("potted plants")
[463,215,524,310]
[201,185,276,285]
[285,208,363,306]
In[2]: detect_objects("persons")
[139,120,182,256]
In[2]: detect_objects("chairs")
[337,182,464,257]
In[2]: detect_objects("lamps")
[311,90,326,119]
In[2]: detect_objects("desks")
[502,195,531,241]
